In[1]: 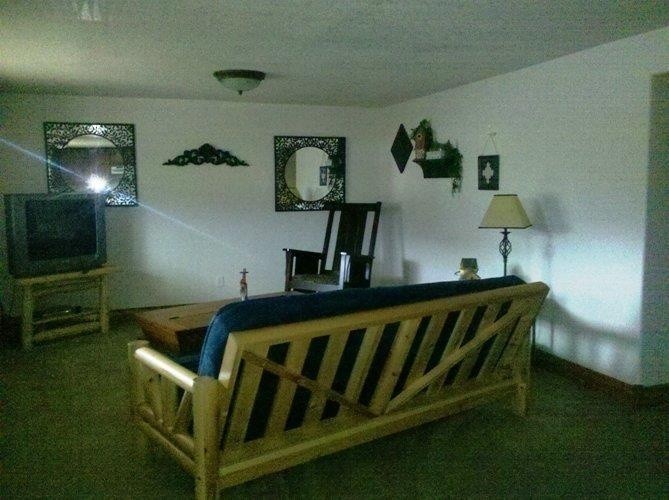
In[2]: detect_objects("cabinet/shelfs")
[11,265,123,350]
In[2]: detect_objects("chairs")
[283,202,383,293]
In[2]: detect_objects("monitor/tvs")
[4,191,108,279]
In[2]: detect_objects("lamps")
[480,194,531,280]
[214,70,266,93]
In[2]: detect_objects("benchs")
[126,281,549,500]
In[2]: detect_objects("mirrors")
[273,135,345,211]
[45,122,137,207]
